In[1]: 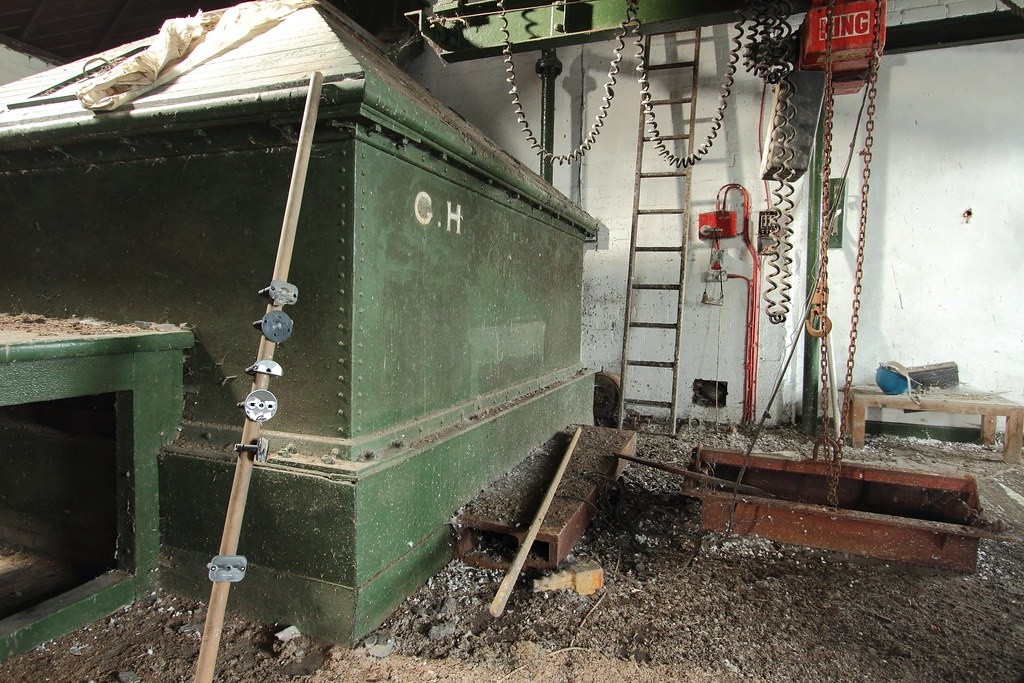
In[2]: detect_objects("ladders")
[616,26,704,438]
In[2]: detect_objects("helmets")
[876,363,923,395]
